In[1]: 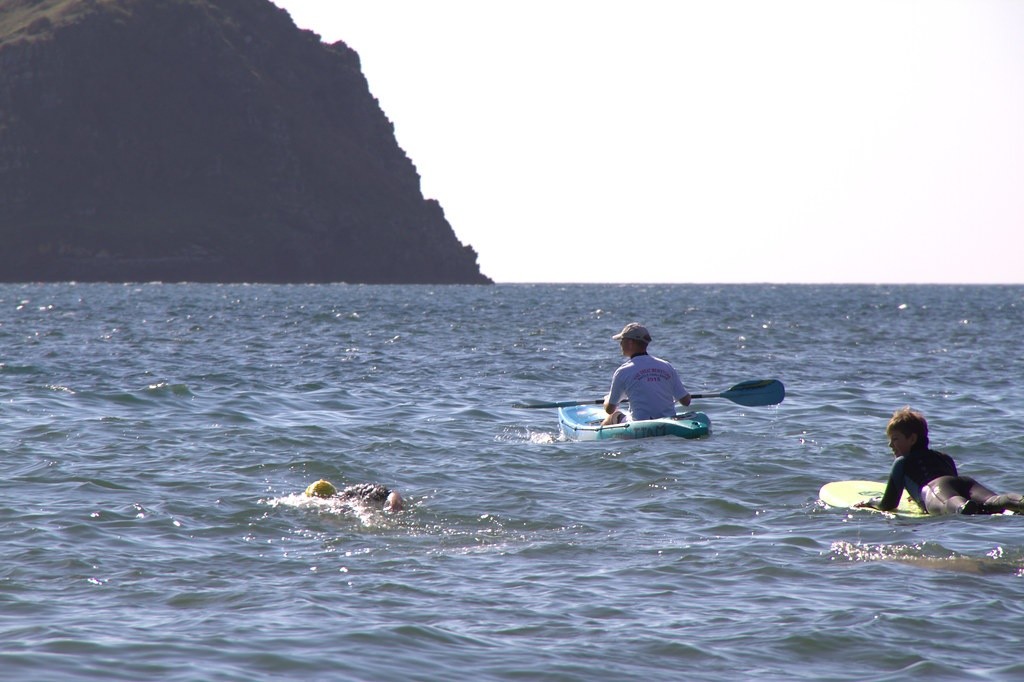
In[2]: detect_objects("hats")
[611,322,651,343]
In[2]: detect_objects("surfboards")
[819,481,1014,517]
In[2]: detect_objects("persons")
[600,322,691,426]
[855,406,1024,516]
[304,478,404,512]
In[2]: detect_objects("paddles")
[512,379,785,409]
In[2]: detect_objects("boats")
[558,403,712,442]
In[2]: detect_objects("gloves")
[854,497,884,510]
